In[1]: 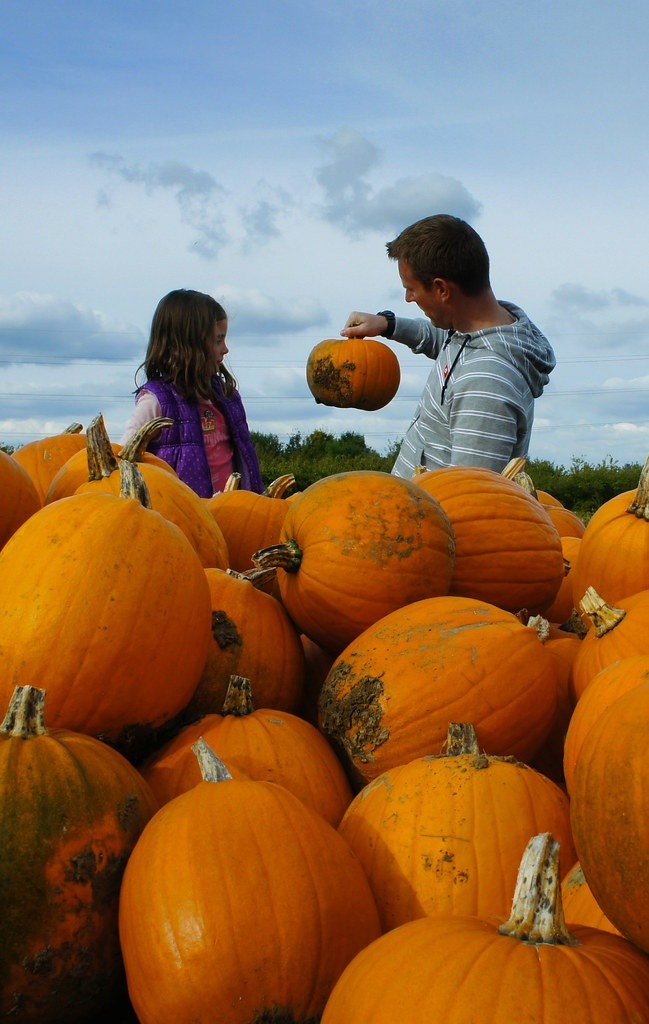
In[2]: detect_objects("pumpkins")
[0,413,649,1024]
[306,335,401,412]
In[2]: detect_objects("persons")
[341,212,557,481]
[121,286,265,498]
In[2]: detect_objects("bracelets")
[378,309,396,338]
